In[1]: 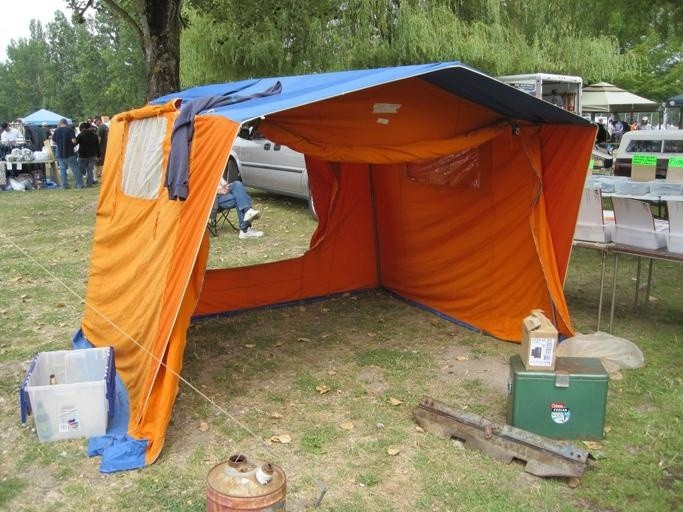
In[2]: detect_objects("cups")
[228,455,246,467]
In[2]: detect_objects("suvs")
[218,119,317,222]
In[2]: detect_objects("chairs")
[206,200,250,241]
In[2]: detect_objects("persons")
[551,88,564,107]
[595,116,652,150]
[0,115,109,189]
[206,155,265,239]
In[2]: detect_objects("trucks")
[491,72,583,116]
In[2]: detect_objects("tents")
[71,60,600,476]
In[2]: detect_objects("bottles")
[254,463,275,485]
[47,374,57,384]
[35,401,52,439]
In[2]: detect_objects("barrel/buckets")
[205,454,288,512]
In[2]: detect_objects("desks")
[3,155,54,183]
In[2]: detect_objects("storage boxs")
[572,155,682,334]
[520,309,560,375]
[18,346,118,444]
[508,355,609,442]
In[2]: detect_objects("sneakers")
[243,208,261,222]
[239,227,264,239]
